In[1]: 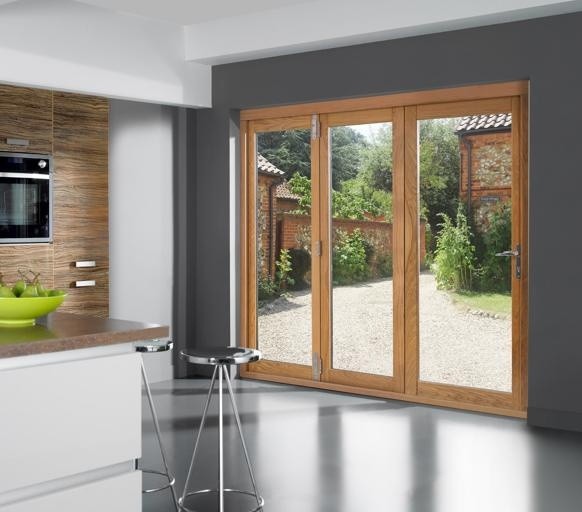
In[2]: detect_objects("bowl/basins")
[0,285,68,328]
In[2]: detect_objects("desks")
[1,310,169,512]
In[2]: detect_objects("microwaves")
[0,151,54,244]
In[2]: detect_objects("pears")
[0,266,61,298]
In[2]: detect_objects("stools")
[135,338,178,511]
[177,346,264,512]
